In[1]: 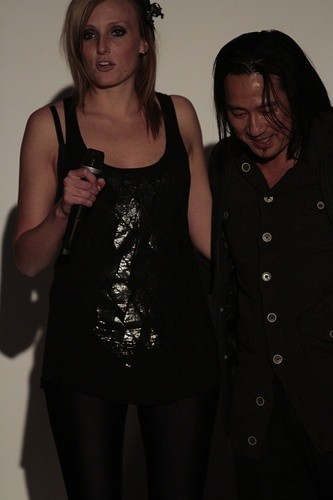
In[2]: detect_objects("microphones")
[63,149,105,255]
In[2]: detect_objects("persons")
[208,30,333,500]
[13,0,212,500]
